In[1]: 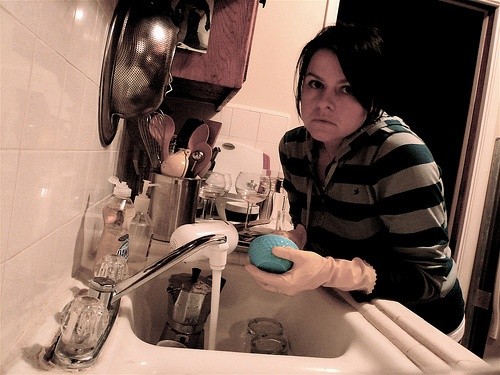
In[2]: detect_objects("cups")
[245,317,288,354]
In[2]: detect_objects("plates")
[157,340,187,348]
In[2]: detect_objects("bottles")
[94,175,135,282]
[129,179,159,264]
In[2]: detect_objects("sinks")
[119,251,375,360]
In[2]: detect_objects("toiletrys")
[96,175,161,276]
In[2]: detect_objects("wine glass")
[235,169,270,231]
[195,172,232,222]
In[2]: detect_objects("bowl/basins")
[98,10,177,147]
[249,234,300,271]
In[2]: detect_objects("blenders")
[158,268,226,349]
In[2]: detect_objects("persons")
[243,23,466,348]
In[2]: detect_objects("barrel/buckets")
[149,170,201,242]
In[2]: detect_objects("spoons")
[187,124,209,152]
[149,114,175,160]
[191,150,205,171]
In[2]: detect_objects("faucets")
[87,234,227,311]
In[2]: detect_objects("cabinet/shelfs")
[168,0,259,110]
